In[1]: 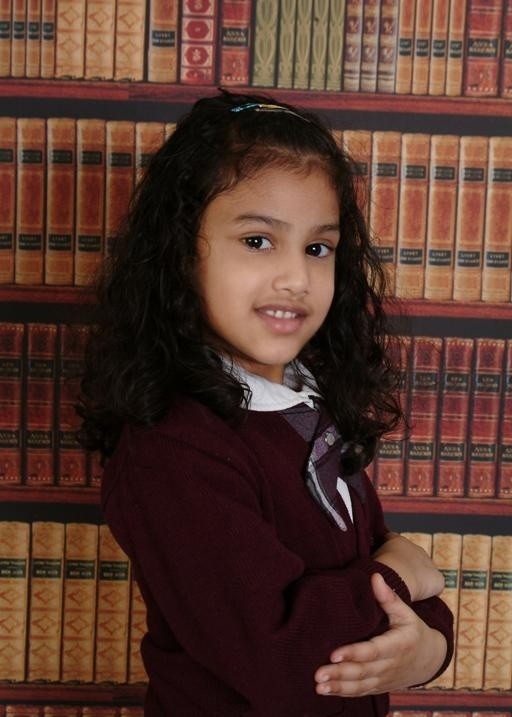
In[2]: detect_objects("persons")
[74,94,456,717]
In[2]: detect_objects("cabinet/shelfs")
[0,76,512,709]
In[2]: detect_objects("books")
[366,338,509,716]
[2,321,150,717]
[3,1,511,303]
